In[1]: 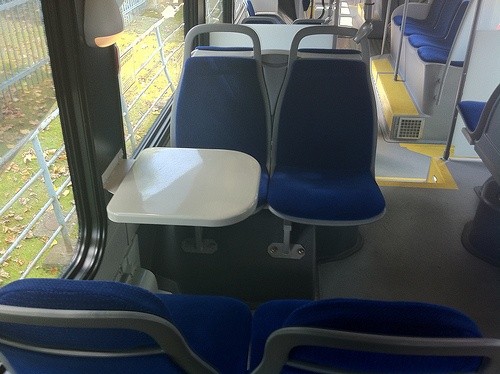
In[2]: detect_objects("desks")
[101,147,261,227]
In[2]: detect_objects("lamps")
[83,0,123,48]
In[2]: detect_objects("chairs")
[391,0,472,67]
[266,25,387,261]
[454,83,499,146]
[254,299,499,374]
[170,24,272,254]
[0,279,252,374]
[242,0,325,25]
